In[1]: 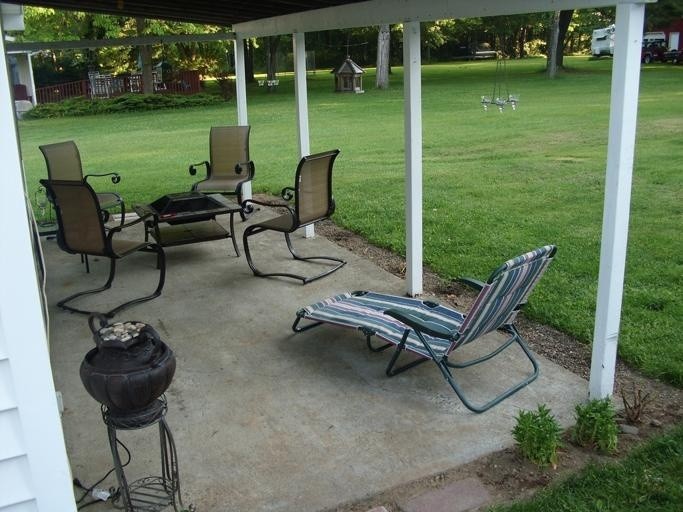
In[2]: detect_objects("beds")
[291,243,557,414]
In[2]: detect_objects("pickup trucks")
[642,41,680,64]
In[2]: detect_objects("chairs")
[188,126,261,222]
[39,140,126,242]
[241,148,347,284]
[39,179,166,319]
